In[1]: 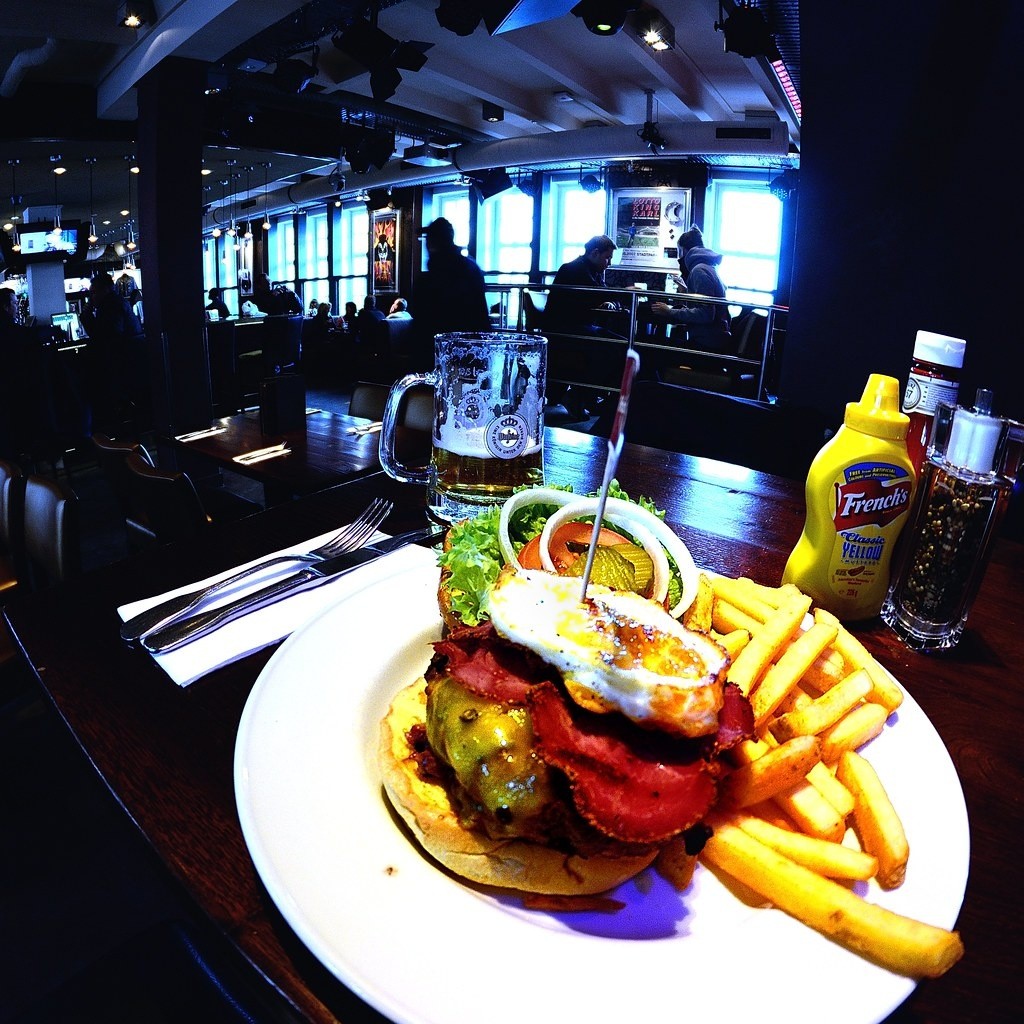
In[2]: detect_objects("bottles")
[780,328,1010,656]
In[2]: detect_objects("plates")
[235,563,970,1024]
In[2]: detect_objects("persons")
[206,288,230,318]
[310,298,319,316]
[311,302,336,336]
[387,298,411,319]
[343,302,357,321]
[646,223,732,380]
[541,237,629,420]
[348,295,385,340]
[416,217,493,373]
[0,289,47,346]
[79,274,144,431]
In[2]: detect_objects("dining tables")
[0,424,1024,1024]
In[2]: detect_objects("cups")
[634,282,648,302]
[342,321,349,331]
[665,273,680,293]
[378,332,549,526]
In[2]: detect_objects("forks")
[119,497,395,641]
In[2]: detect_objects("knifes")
[141,525,448,655]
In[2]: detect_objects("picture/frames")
[374,213,397,290]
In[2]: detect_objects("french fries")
[525,575,964,974]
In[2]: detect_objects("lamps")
[268,1,799,206]
[116,0,158,29]
[7,154,271,257]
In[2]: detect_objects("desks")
[39,315,307,425]
[170,406,382,511]
[589,307,668,389]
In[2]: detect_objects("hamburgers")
[377,479,757,896]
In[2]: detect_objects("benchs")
[378,319,414,387]
[302,315,348,377]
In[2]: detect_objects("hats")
[677,223,704,249]
[417,217,453,236]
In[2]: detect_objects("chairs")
[348,380,392,423]
[1,432,264,775]
[524,291,571,407]
[403,388,434,433]
[662,312,758,395]
